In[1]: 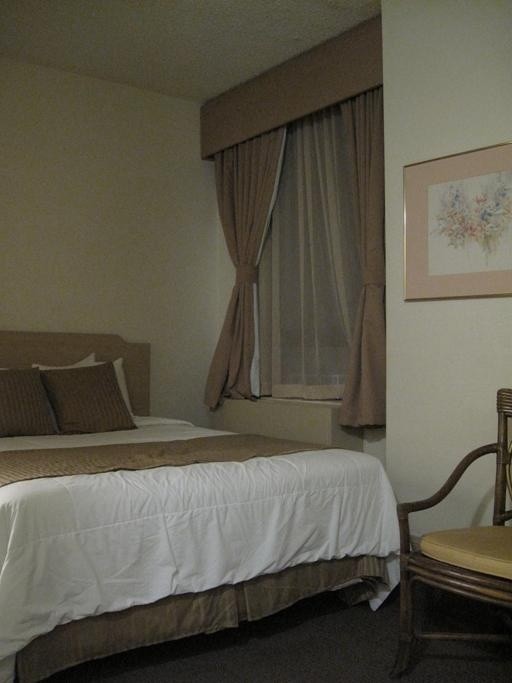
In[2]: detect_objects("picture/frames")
[402,139,511,301]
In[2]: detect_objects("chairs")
[389,388,512,681]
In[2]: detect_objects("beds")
[0,329,384,683]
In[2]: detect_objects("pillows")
[1,352,138,436]
